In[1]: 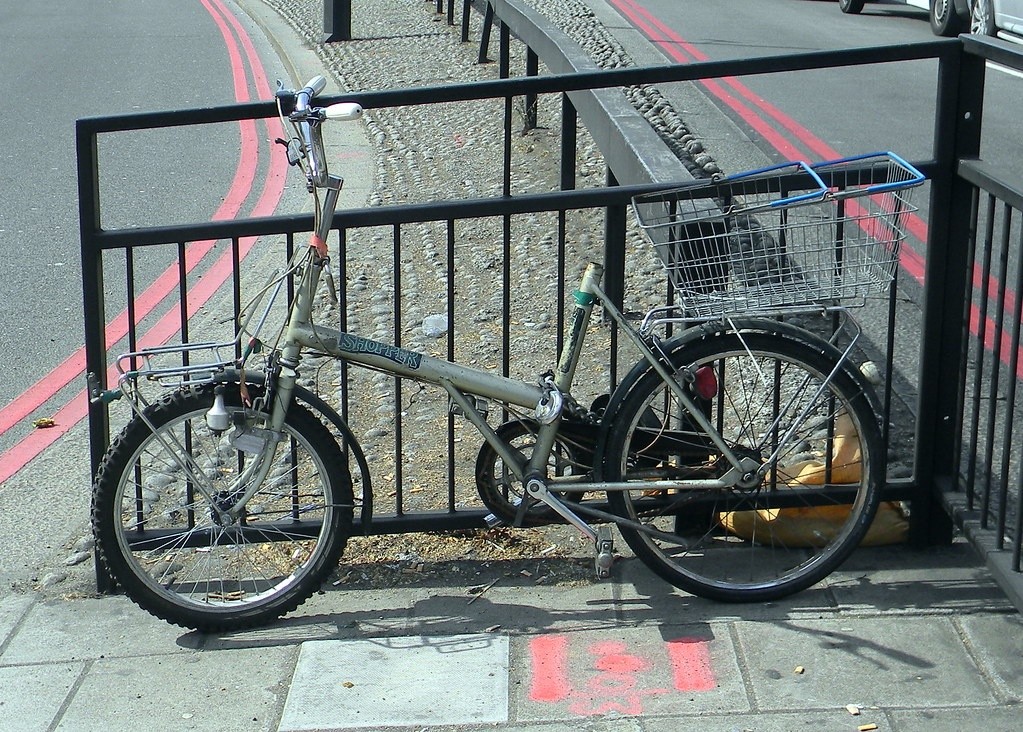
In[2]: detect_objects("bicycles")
[83,72,926,633]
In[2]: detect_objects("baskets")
[630,150,926,317]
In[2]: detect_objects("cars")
[838,0,977,37]
[964,0,1023,49]
